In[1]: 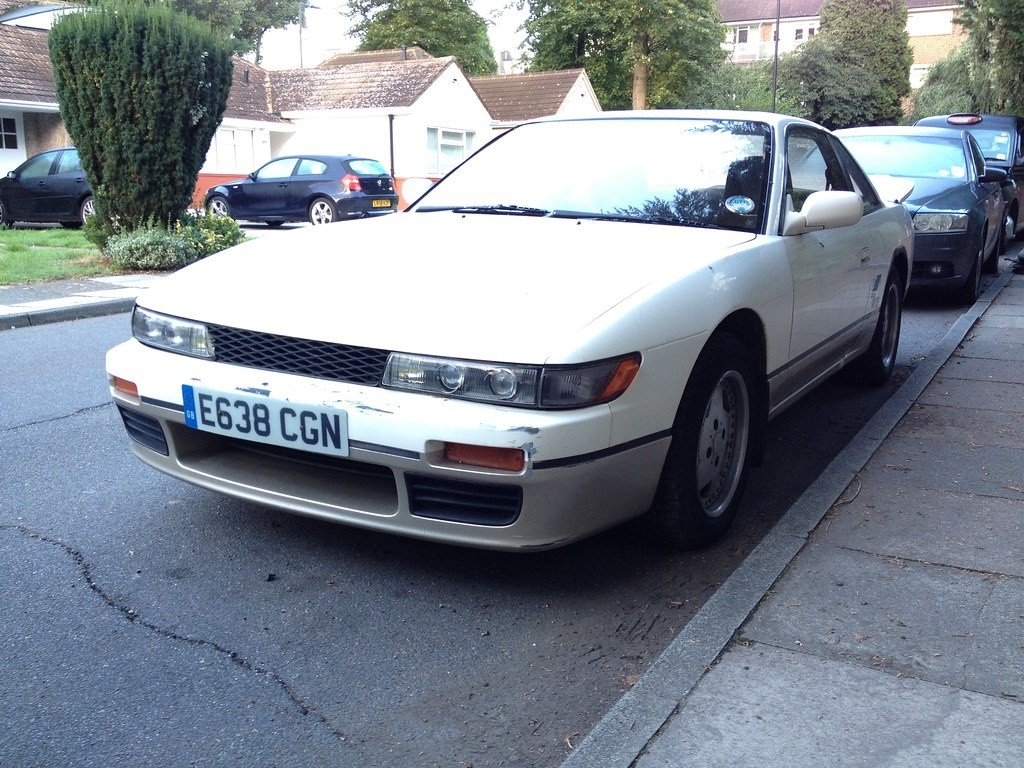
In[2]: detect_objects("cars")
[101,108,915,556]
[829,125,1007,309]
[907,113,1024,255]
[203,153,400,227]
[0,147,101,232]
